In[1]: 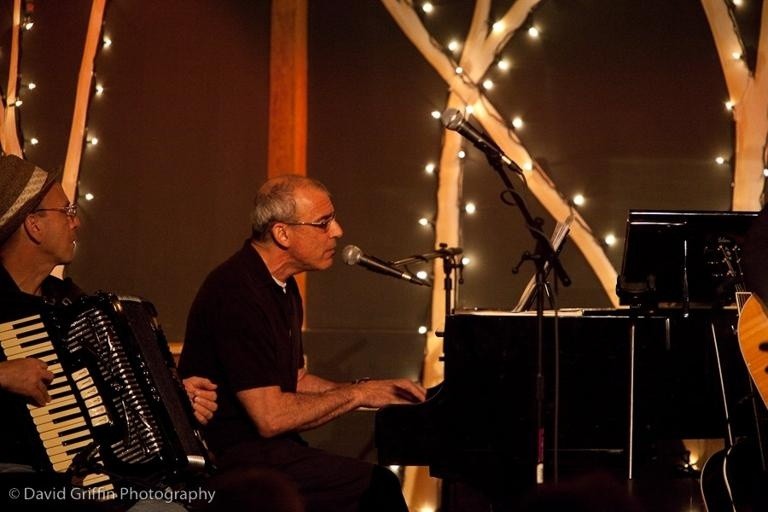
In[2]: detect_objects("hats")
[1,155,62,243]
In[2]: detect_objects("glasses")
[29,204,77,217]
[282,210,335,233]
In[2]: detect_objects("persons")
[176,176,427,512]
[0,154,219,512]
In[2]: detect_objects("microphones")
[442,107,523,174]
[342,245,424,285]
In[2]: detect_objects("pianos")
[373,307,764,507]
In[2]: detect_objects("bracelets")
[352,377,371,384]
